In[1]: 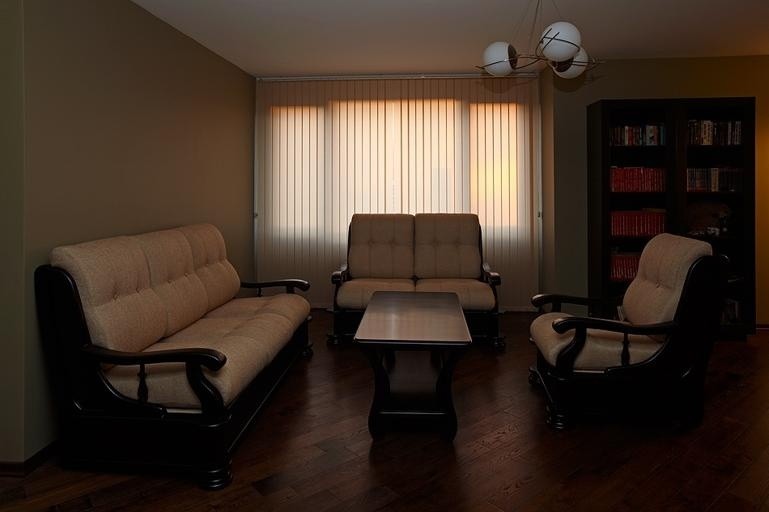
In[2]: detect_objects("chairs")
[528,232,730,431]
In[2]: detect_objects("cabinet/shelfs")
[585,97,756,341]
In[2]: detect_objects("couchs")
[325,213,506,355]
[34,221,314,490]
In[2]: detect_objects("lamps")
[475,1,605,81]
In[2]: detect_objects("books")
[611,119,742,281]
